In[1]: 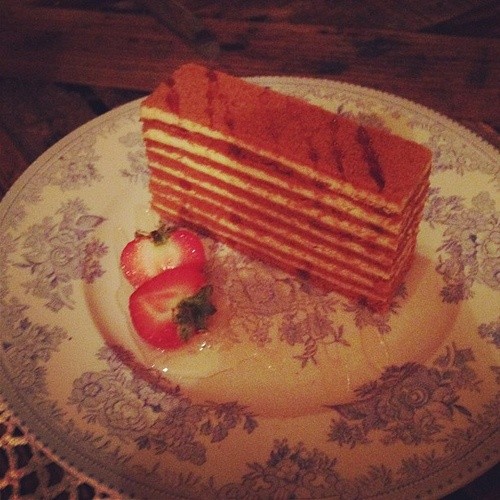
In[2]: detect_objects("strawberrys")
[118,224,215,351]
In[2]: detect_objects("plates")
[0,76,500,500]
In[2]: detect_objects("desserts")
[137,63,435,314]
